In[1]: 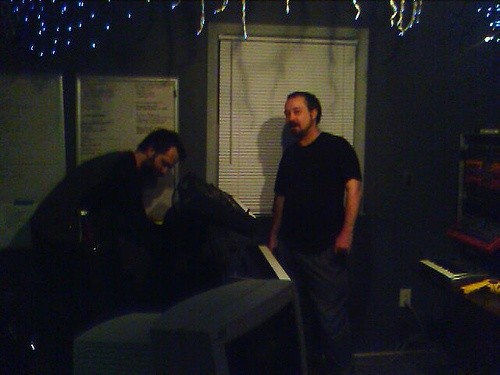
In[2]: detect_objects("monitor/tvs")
[73,279,307,375]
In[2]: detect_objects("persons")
[27,128,187,321]
[268,91,361,375]
